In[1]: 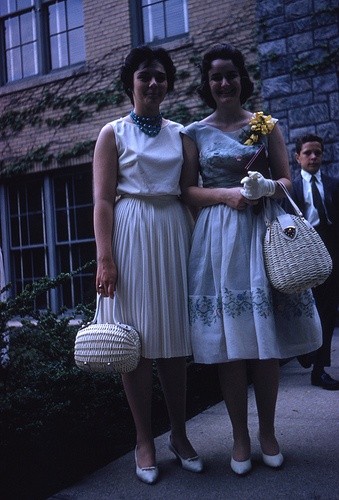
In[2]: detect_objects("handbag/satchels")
[263,179,333,296]
[73,289,141,375]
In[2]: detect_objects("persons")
[179,42,323,475]
[93,45,204,485]
[283,134,339,390]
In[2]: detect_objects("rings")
[99,285,102,288]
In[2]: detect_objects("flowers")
[238,111,275,147]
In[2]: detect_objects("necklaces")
[129,110,162,137]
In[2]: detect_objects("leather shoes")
[299,353,312,368]
[311,370,339,390]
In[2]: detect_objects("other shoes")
[231,436,252,475]
[257,431,284,468]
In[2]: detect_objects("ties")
[311,177,328,226]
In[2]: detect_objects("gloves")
[240,171,276,200]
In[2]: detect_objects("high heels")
[168,434,204,473]
[135,443,159,486]
